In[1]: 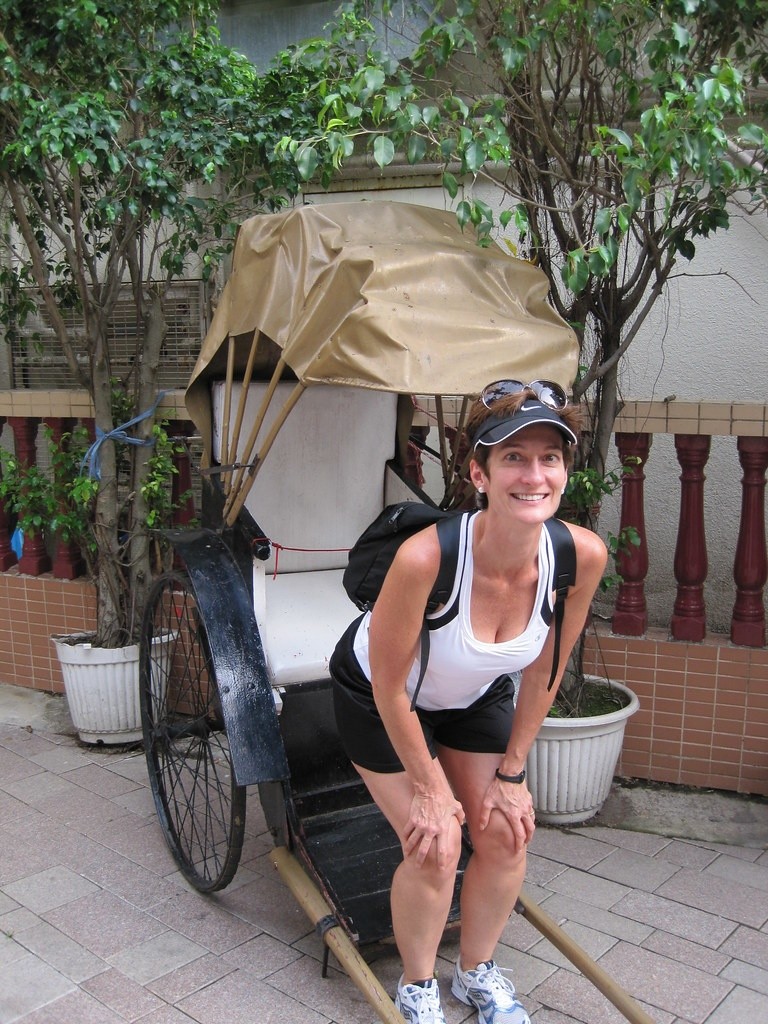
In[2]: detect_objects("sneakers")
[394,973,447,1024]
[451,954,531,1024]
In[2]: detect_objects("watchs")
[495,768,525,784]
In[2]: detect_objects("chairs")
[212,377,401,688]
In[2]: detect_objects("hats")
[469,399,578,448]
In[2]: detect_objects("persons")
[328,385,606,1024]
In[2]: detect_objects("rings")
[530,810,535,815]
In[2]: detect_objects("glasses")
[477,378,569,414]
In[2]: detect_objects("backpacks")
[343,500,577,613]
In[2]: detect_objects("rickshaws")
[136,200,666,1024]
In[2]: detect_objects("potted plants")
[0,0,768,830]
